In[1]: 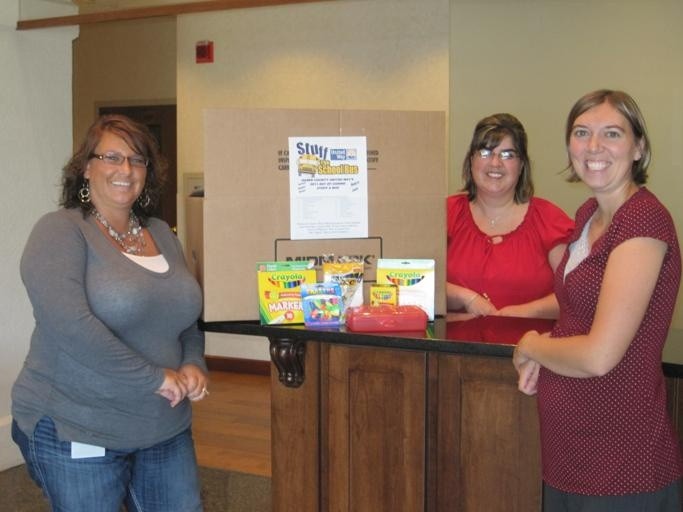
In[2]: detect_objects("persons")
[11,113,210,512]
[511,88,683,512]
[446,112,576,322]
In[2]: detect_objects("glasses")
[474,148,517,160]
[88,151,150,168]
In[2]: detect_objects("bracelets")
[466,294,478,304]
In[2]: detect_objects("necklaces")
[477,205,506,229]
[89,206,147,254]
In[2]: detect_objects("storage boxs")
[184,107,447,325]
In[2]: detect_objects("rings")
[203,389,209,394]
